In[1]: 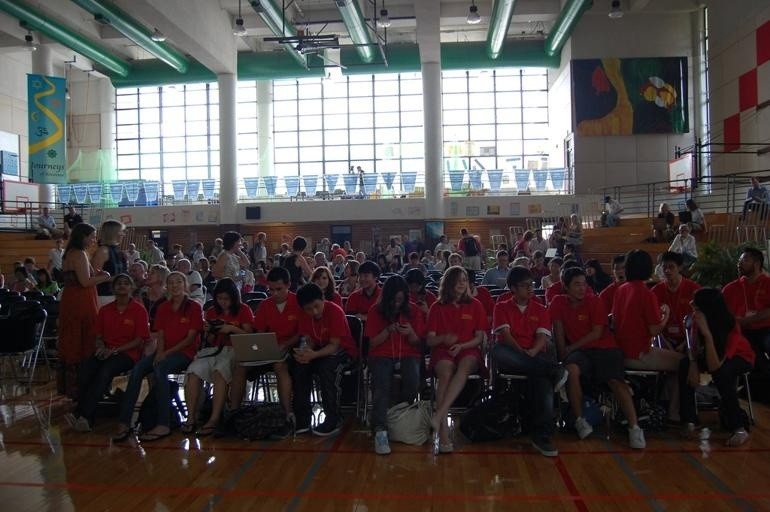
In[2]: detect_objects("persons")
[738,176,770,221]
[63,205,84,239]
[357,165,366,199]
[0,217,769,457]
[601,195,624,228]
[36,207,65,239]
[650,202,676,240]
[678,199,708,234]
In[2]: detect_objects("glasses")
[517,281,536,289]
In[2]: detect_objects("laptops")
[229,332,289,364]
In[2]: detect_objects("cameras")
[208,319,225,332]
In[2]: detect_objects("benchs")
[574,206,769,276]
[0,231,99,277]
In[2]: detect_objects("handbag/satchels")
[614,405,665,435]
[385,400,433,448]
[52,266,78,289]
[458,395,524,442]
[233,404,285,442]
[137,380,180,432]
[95,388,125,418]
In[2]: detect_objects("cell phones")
[397,324,408,334]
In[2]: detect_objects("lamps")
[375,0,391,29]
[608,0,625,20]
[465,0,482,26]
[19,30,38,54]
[65,88,71,100]
[230,0,247,38]
[150,26,167,43]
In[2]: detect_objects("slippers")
[182,422,194,434]
[138,430,171,442]
[113,428,130,442]
[196,424,215,436]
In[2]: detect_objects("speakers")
[246,206,260,219]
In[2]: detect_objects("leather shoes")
[429,418,454,454]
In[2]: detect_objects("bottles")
[298,335,309,352]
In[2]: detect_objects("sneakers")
[575,417,593,440]
[532,442,558,457]
[629,428,646,449]
[295,423,310,435]
[726,430,748,447]
[64,410,91,432]
[666,417,700,432]
[312,416,344,437]
[554,368,568,392]
[374,430,391,456]
